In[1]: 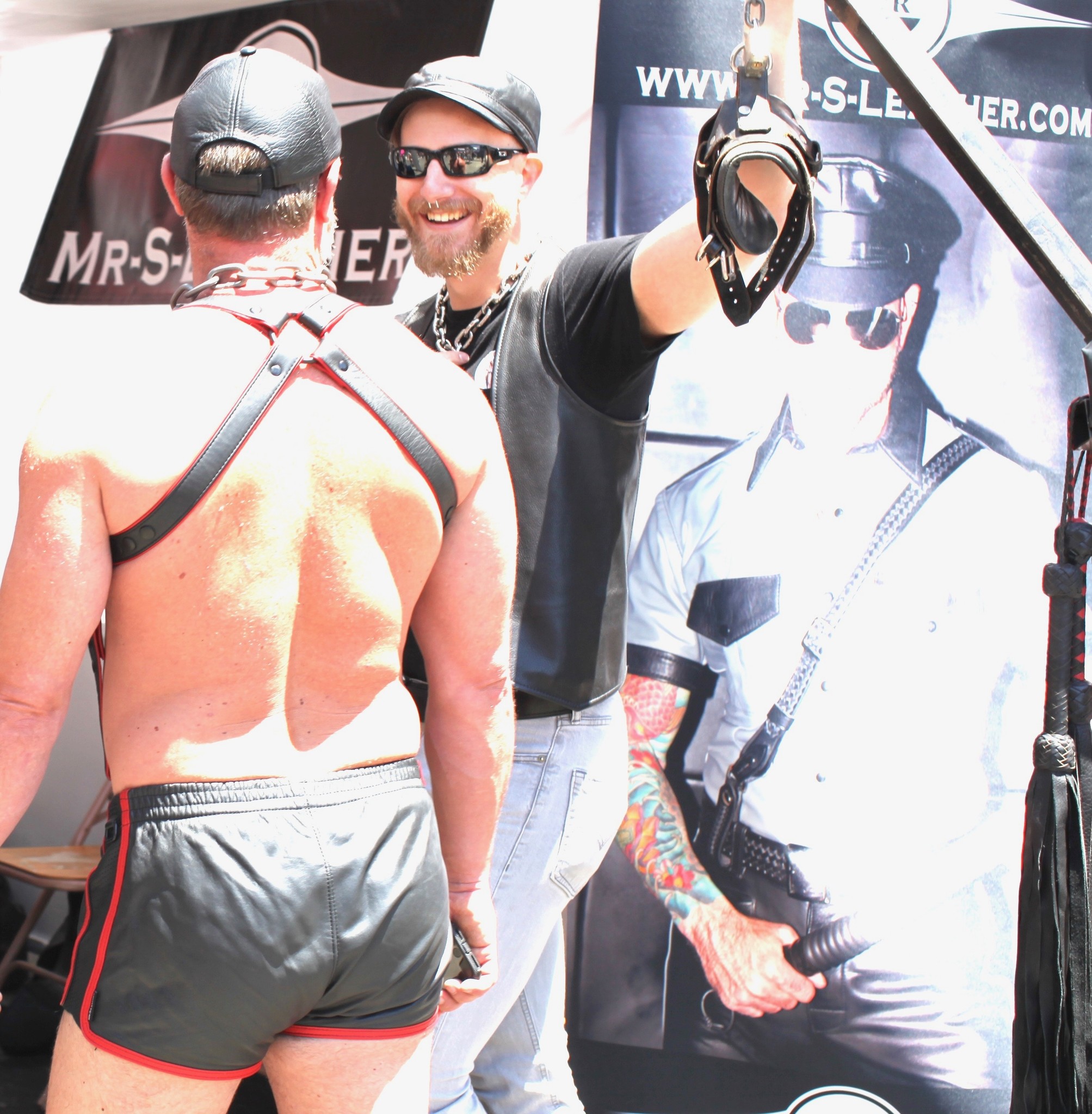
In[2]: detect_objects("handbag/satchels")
[577,809,756,1056]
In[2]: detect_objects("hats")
[376,56,541,155]
[170,45,343,196]
[797,154,963,270]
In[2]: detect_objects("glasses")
[388,142,527,178]
[777,301,905,350]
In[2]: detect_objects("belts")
[402,678,572,723]
[727,823,830,905]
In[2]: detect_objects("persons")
[0,49,518,1114]
[374,0,804,1114]
[622,149,1062,1114]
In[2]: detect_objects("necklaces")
[432,252,527,364]
[168,262,338,305]
